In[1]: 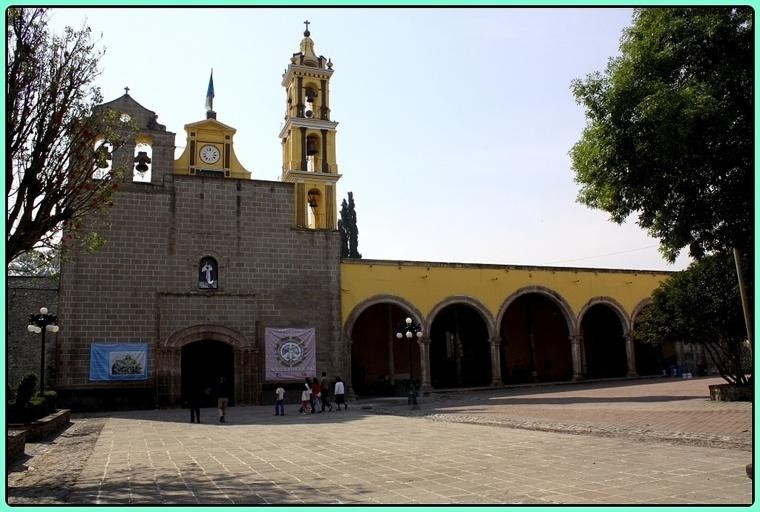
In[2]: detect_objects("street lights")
[396,317,423,406]
[27,307,60,393]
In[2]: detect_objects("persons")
[183,373,208,424]
[311,378,322,413]
[297,377,316,414]
[208,372,231,424]
[333,375,348,411]
[320,371,333,412]
[272,381,287,417]
[300,387,313,416]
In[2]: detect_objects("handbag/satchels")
[314,391,322,397]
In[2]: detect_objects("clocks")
[199,144,221,165]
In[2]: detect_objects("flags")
[205,75,214,109]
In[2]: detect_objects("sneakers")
[190,403,349,424]
